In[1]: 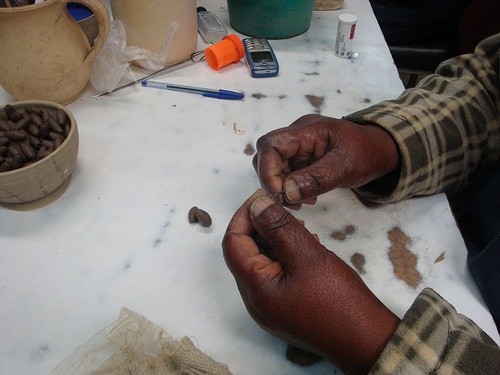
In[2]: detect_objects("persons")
[219,24,500,374]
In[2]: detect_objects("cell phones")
[241,36,281,78]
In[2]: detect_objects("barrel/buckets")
[227,0,315,40]
[110,0,198,69]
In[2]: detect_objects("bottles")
[196,6,228,43]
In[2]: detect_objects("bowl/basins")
[1,100,79,212]
[77,13,99,49]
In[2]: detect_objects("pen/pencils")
[140,80,245,102]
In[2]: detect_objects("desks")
[0,0,497,375]
